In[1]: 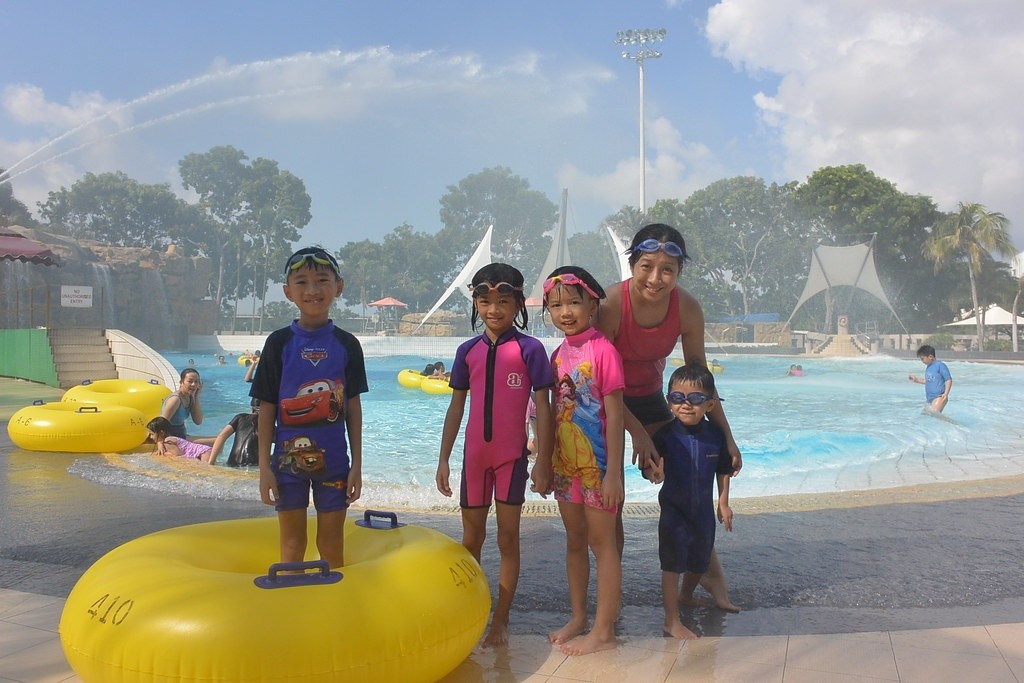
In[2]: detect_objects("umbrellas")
[0,228,60,267]
[367,296,407,306]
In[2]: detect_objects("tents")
[941,306,1024,326]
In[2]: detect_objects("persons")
[712,358,724,371]
[592,223,742,622]
[642,363,733,640]
[530,266,624,656]
[208,397,278,467]
[408,361,451,380]
[434,264,553,648]
[247,248,369,575]
[909,345,953,414]
[161,368,224,455]
[147,417,213,462]
[786,364,804,377]
[187,347,262,383]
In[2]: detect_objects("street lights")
[615,27,667,221]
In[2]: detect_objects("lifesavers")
[706,362,721,373]
[397,368,455,394]
[238,356,260,365]
[58,513,494,683]
[7,377,174,454]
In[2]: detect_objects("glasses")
[474,282,514,296]
[637,240,682,257]
[289,252,330,270]
[543,274,579,293]
[671,392,705,406]
